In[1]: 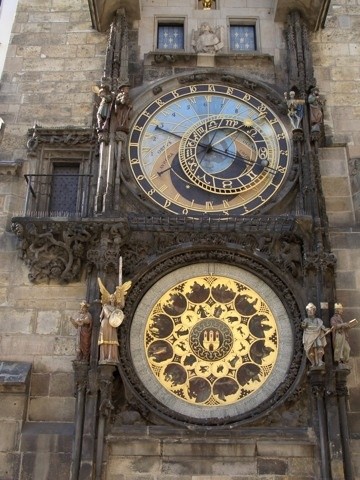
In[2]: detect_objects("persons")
[68,301,92,362]
[97,294,126,365]
[96,84,115,132]
[329,303,353,370]
[302,303,330,369]
[115,84,132,134]
[191,22,222,55]
[308,87,327,133]
[283,91,304,129]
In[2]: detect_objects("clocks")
[129,262,293,421]
[125,83,292,220]
